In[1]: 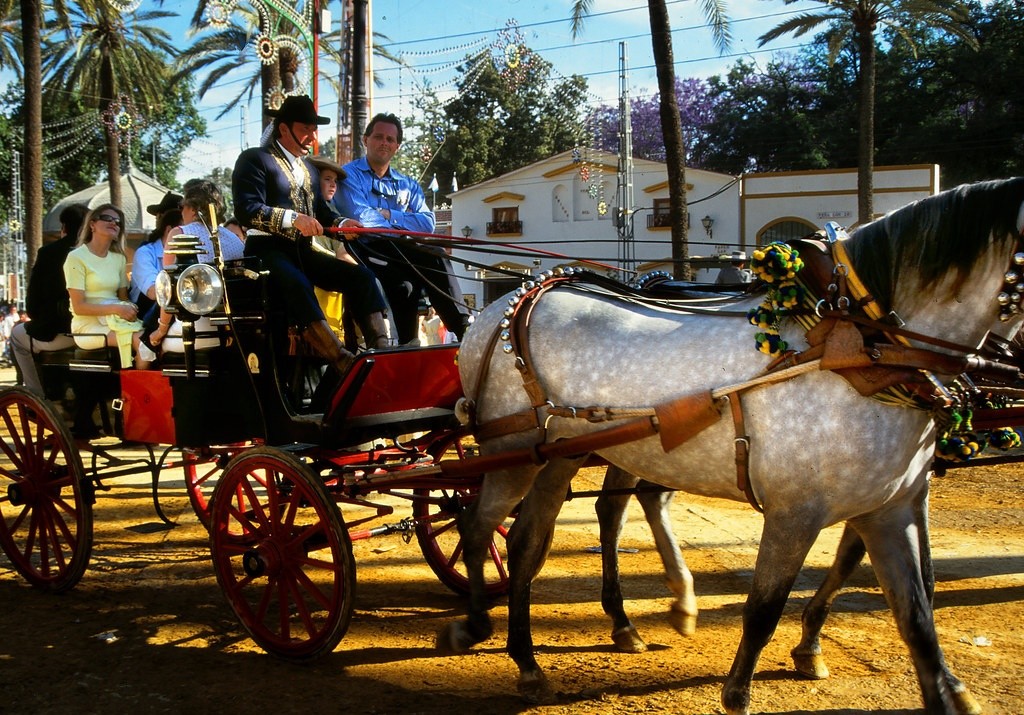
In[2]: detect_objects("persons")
[715,251,752,284]
[231,95,476,375]
[0,179,247,418]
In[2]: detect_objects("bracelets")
[376,207,382,213]
[158,318,169,326]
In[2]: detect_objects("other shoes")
[306,155,347,180]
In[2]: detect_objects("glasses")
[93,214,123,227]
[371,172,398,198]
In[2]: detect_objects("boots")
[356,312,421,350]
[302,319,355,373]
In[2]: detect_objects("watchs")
[291,211,298,224]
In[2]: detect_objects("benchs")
[29,330,223,425]
[219,244,392,351]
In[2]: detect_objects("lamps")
[154,263,224,323]
[462,225,472,238]
[701,215,714,239]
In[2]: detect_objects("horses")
[435,175,1024,715]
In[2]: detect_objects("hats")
[262,96,331,124]
[146,191,184,216]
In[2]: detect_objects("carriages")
[0,171,1024,715]
